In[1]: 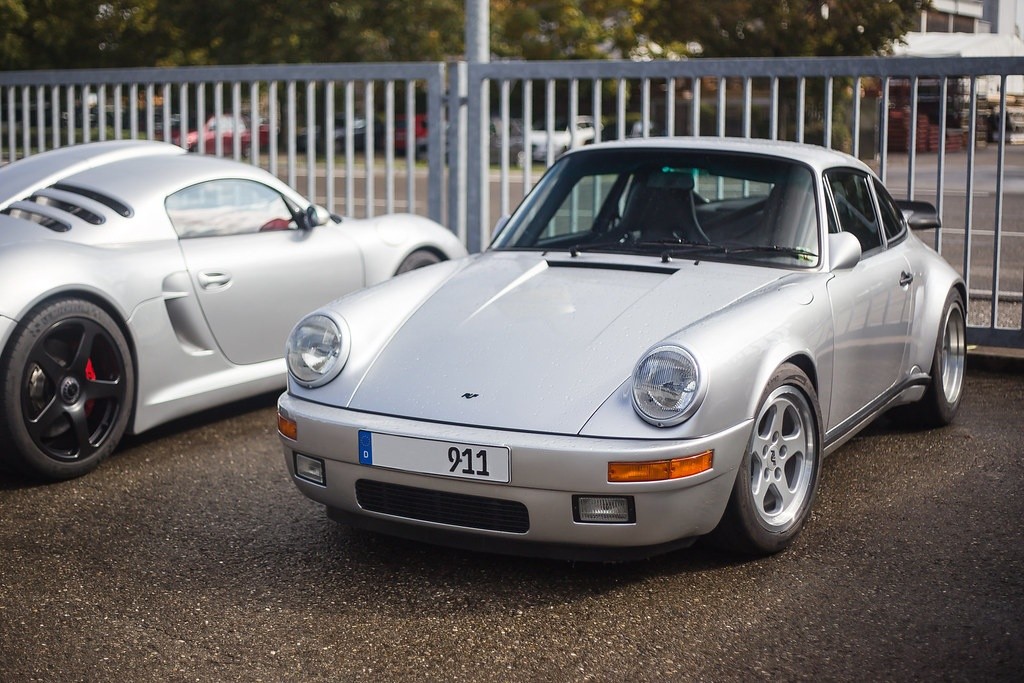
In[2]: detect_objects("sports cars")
[0,140,471,483]
[276,135,970,565]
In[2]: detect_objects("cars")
[158,110,660,166]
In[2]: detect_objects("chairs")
[740,180,837,249]
[595,173,711,244]
